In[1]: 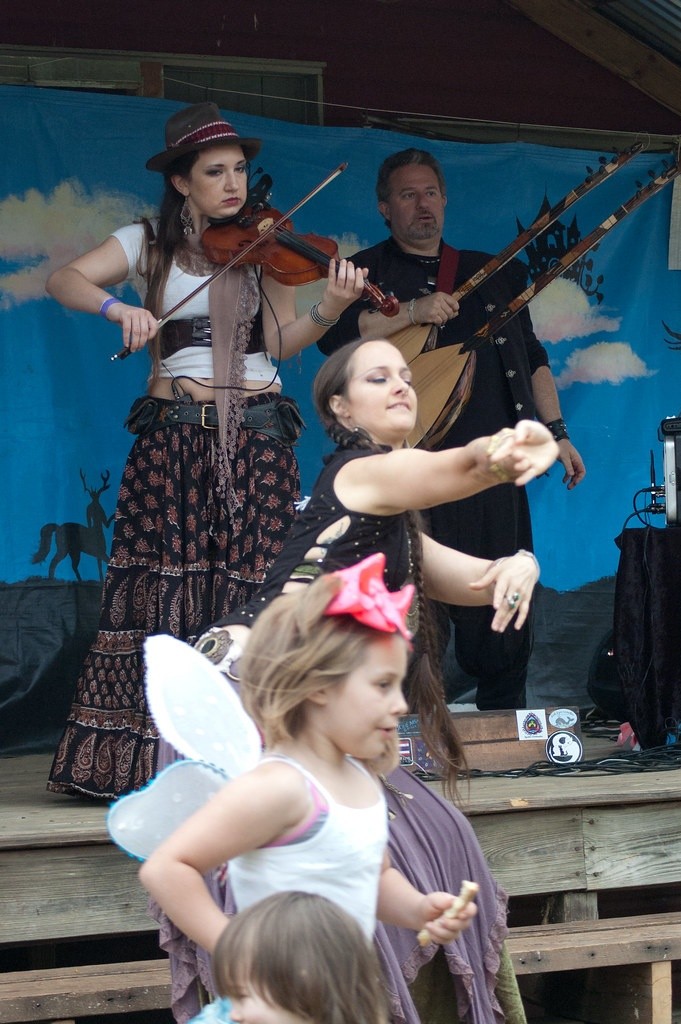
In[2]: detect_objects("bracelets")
[98,297,122,321]
[309,301,341,328]
[485,428,515,484]
[546,413,569,442]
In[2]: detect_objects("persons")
[212,888,398,1024]
[149,336,559,1024]
[44,103,369,800]
[316,147,586,710]
[140,554,479,1024]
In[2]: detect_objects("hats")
[146,102,262,173]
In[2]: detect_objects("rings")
[507,593,519,608]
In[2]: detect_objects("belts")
[138,396,298,446]
[193,627,242,681]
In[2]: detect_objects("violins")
[201,201,400,318]
